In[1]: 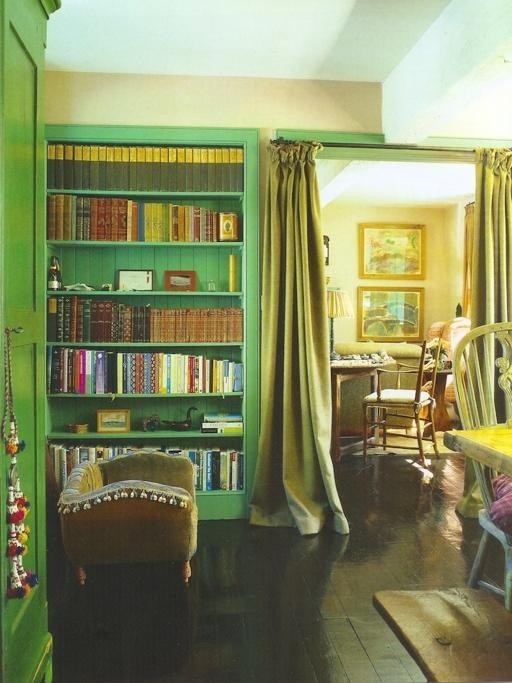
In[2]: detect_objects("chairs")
[451,318,510,611]
[361,339,444,470]
[56,447,200,588]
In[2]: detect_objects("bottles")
[208,280,215,292]
[47,255,62,290]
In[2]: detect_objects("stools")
[371,588,512,683]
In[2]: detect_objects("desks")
[330,355,397,461]
[441,424,510,480]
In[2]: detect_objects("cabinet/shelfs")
[0,0,58,682]
[41,126,265,524]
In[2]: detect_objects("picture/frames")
[356,218,427,343]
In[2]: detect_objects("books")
[229,255,236,293]
[50,347,242,394]
[201,413,243,433]
[48,146,244,242]
[50,296,243,344]
[50,443,243,490]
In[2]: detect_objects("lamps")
[325,286,348,360]
